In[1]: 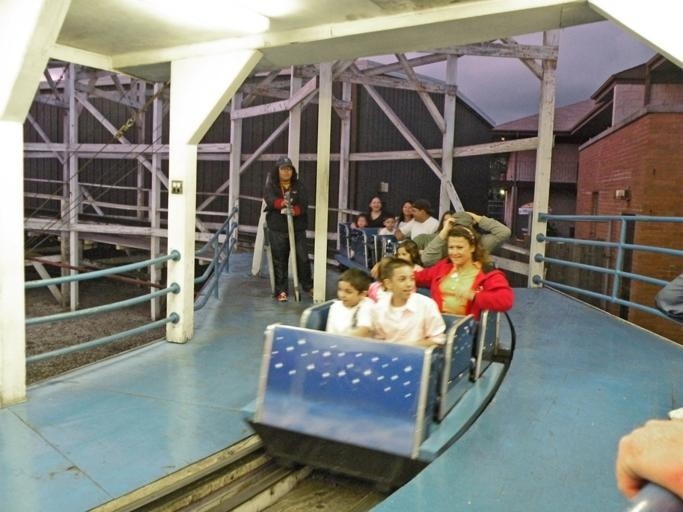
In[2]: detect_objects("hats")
[277,155,293,169]
[449,211,475,228]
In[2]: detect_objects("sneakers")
[307,288,313,298]
[277,290,288,302]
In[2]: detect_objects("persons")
[348,192,391,260]
[355,214,370,229]
[652,269,682,324]
[413,224,514,321]
[261,156,313,302]
[370,240,423,279]
[377,214,398,236]
[411,210,455,250]
[614,404,682,502]
[323,268,375,338]
[393,200,416,239]
[372,257,446,348]
[421,209,512,267]
[394,199,442,240]
